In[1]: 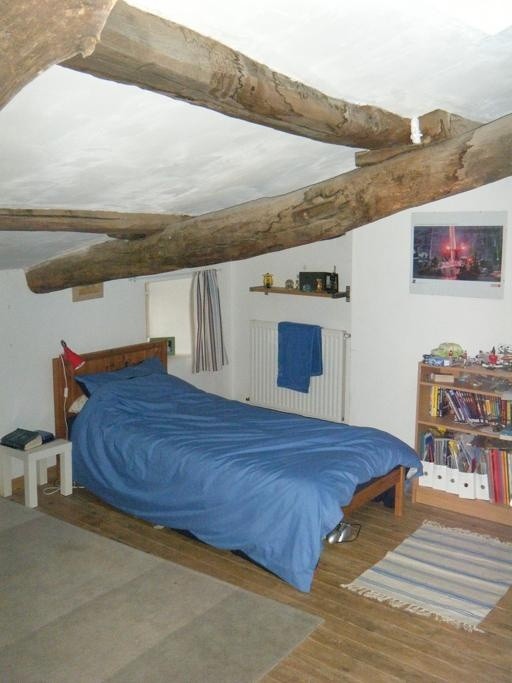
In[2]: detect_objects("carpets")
[339,520,512,634]
[0,497,326,683]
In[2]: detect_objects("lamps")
[57,342,87,441]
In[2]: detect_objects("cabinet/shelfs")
[411,362,512,528]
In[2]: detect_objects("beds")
[52,339,421,593]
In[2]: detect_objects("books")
[32,430,55,444]
[0,427,43,451]
[417,341,512,507]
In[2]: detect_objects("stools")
[0,438,73,509]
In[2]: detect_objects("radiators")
[242,320,351,423]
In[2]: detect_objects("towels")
[277,322,322,393]
